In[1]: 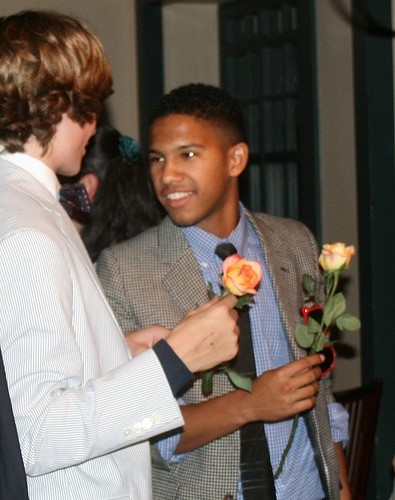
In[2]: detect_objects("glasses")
[300,304,337,377]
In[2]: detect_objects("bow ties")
[58,184,97,223]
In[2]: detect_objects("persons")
[0,11,240,500]
[92,84,352,499]
[61,126,164,261]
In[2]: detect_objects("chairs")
[327,381,382,500]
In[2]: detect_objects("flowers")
[200,253,264,395]
[273,241,361,478]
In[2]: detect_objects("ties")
[214,242,277,500]
